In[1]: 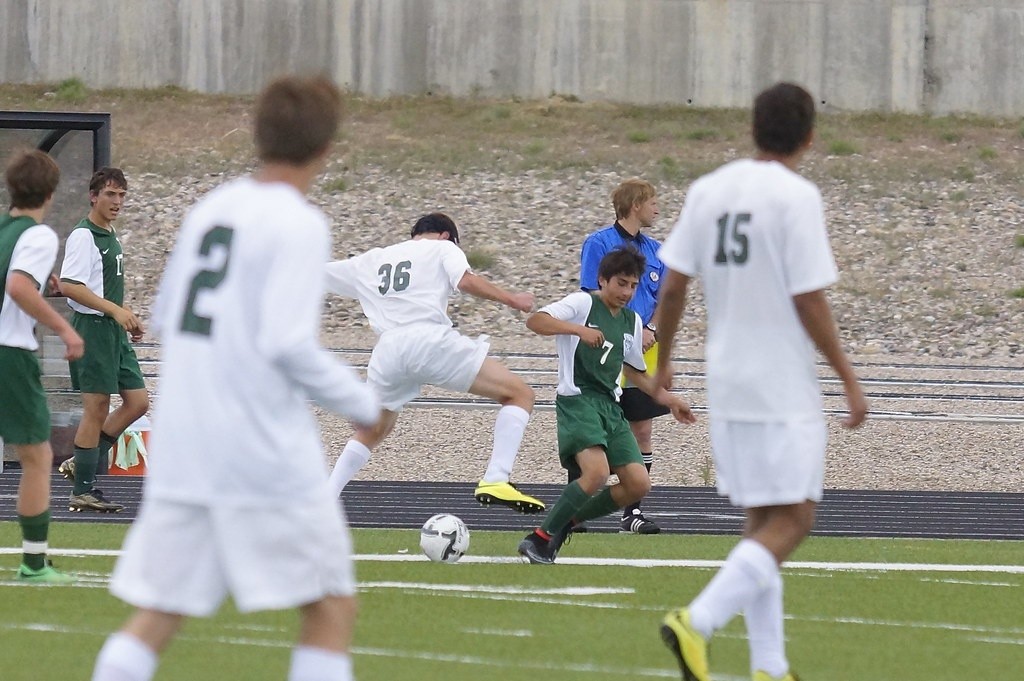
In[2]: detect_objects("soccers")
[420,512,470,564]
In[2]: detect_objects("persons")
[55,165,150,511]
[316,213,546,522]
[87,75,392,680]
[0,146,87,588]
[518,237,698,564]
[573,176,679,536]
[656,82,872,681]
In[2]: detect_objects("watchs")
[645,323,657,332]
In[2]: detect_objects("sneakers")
[58,456,77,481]
[546,521,587,564]
[475,479,545,515]
[16,559,77,583]
[752,671,794,681]
[661,606,712,681]
[619,508,660,533]
[68,488,124,513]
[517,533,554,565]
